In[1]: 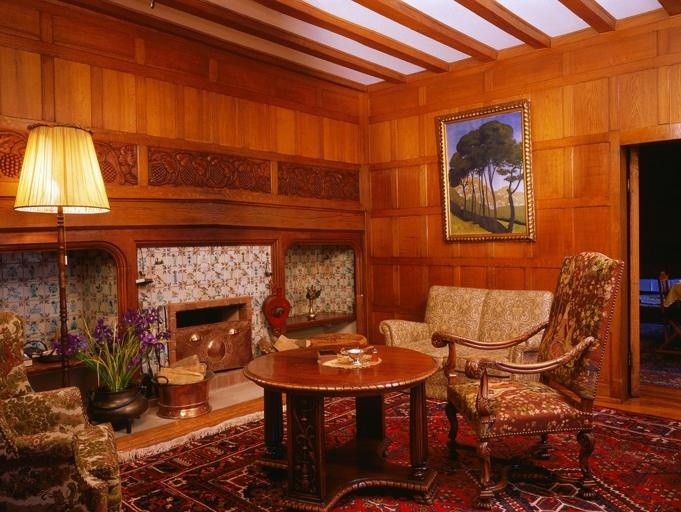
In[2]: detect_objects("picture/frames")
[431,96,538,245]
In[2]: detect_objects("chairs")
[430,247,627,511]
[655,267,680,357]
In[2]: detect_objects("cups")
[346,348,364,366]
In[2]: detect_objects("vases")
[306,297,316,321]
[86,379,150,435]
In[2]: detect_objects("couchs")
[0,300,124,512]
[377,281,555,403]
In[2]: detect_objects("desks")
[241,341,444,511]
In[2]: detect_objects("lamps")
[11,117,112,386]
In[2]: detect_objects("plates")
[36,356,71,363]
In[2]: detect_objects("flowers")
[48,306,175,390]
[304,282,323,303]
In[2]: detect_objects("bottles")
[370,347,379,362]
[658,270,668,297]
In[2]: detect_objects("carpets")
[118,374,680,511]
